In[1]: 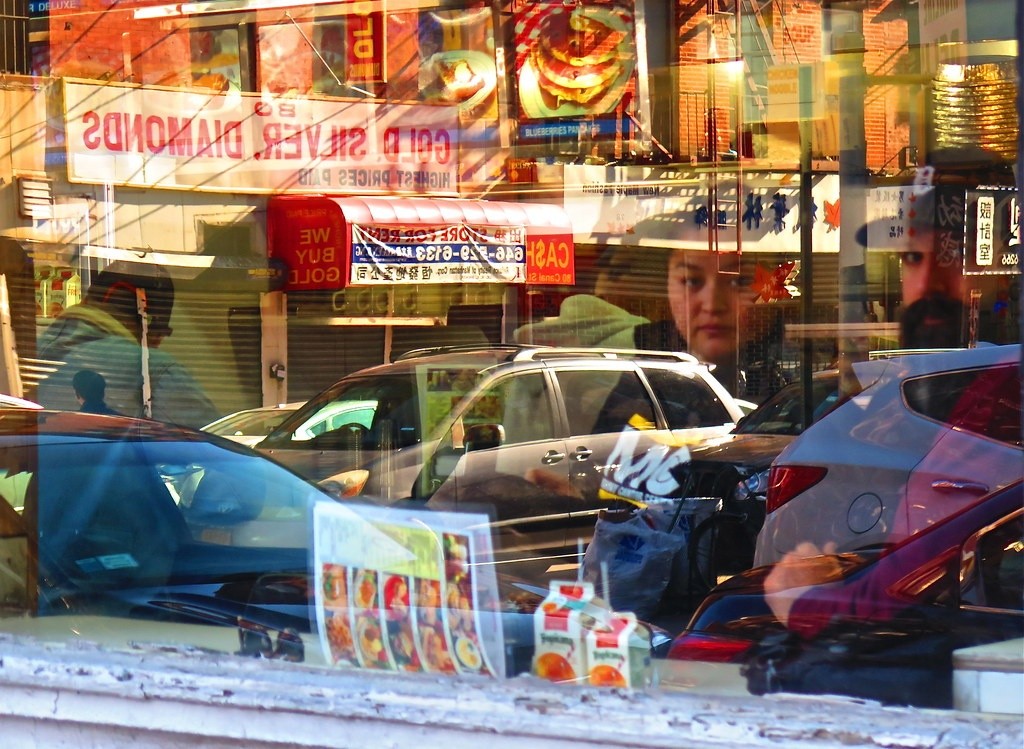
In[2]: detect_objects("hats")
[855,145,1024,249]
[85,257,175,336]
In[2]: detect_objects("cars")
[666,478,1024,710]
[751,347,1024,563]
[599,368,848,507]
[198,398,378,448]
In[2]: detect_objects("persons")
[592,197,791,432]
[71,370,120,415]
[854,147,1024,349]
[34,261,262,542]
[505,254,652,444]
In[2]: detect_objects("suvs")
[1,392,676,680]
[251,343,744,582]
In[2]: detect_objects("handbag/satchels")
[738,620,955,709]
[577,507,690,621]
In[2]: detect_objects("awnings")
[264,194,528,292]
